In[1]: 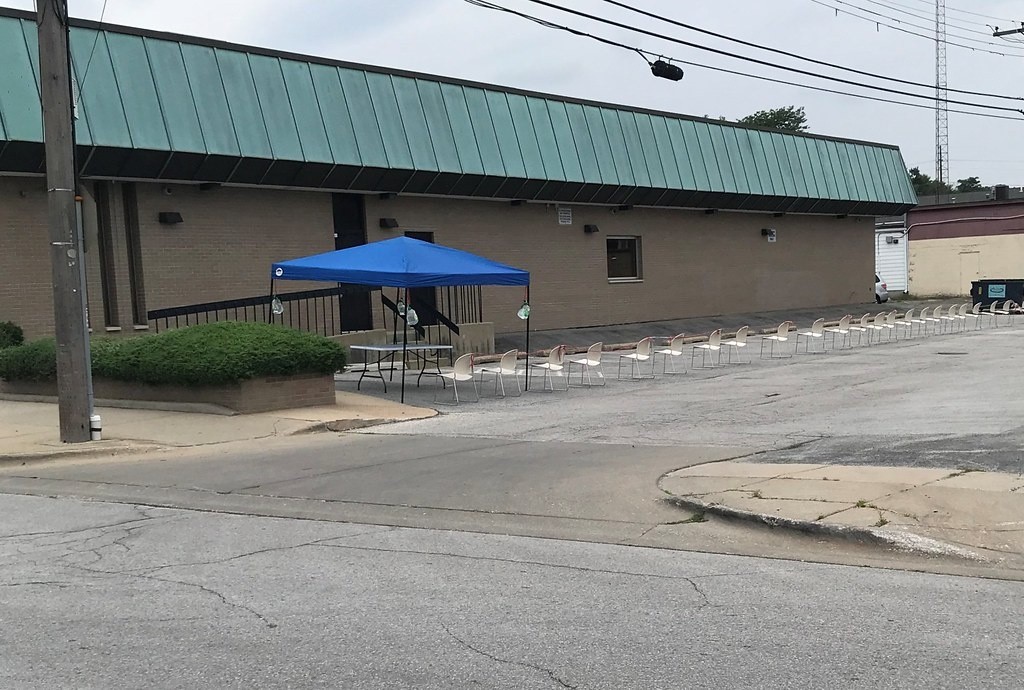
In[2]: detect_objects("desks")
[350,343,453,393]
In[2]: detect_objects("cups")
[90,415,102,440]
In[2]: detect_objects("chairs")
[795,299,1024,355]
[654,334,686,375]
[720,326,751,364]
[759,320,792,359]
[567,342,605,387]
[479,349,522,400]
[618,337,653,382]
[435,353,479,405]
[691,329,723,369]
[529,344,568,392]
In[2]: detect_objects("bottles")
[517,302,530,320]
[407,308,418,325]
[272,297,284,314]
[397,301,405,315]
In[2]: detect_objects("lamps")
[761,228,774,237]
[583,225,600,233]
[159,211,184,224]
[379,217,400,229]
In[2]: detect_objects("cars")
[875,272,889,304]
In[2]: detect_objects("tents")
[269,236,530,403]
